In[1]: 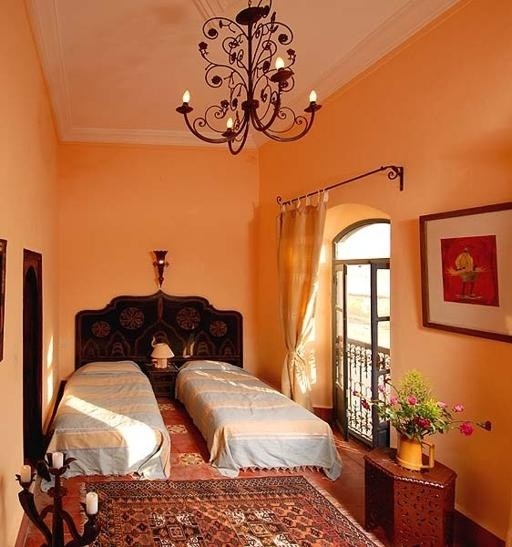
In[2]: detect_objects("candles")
[51,449,65,469]
[85,490,99,516]
[20,461,33,484]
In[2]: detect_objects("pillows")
[70,359,143,374]
[177,358,242,375]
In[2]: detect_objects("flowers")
[346,365,494,444]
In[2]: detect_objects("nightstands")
[143,362,179,401]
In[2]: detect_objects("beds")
[169,353,349,483]
[35,353,174,487]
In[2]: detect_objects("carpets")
[74,465,397,547]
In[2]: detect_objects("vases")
[390,424,437,473]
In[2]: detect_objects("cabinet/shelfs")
[359,443,460,546]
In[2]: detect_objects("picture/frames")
[412,201,512,345]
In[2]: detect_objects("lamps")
[149,341,177,369]
[151,248,171,288]
[166,0,326,159]
